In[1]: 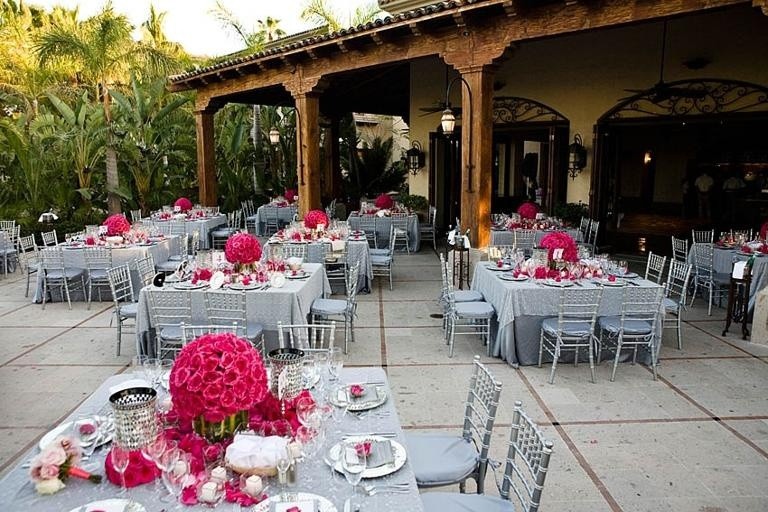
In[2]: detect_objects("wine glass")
[178,242,298,285]
[270,194,412,242]
[501,246,627,287]
[150,205,220,221]
[61,222,159,244]
[72,347,369,512]
[720,230,765,255]
[492,212,564,231]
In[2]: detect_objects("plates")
[611,270,639,278]
[486,263,528,281]
[174,283,206,290]
[210,271,224,289]
[63,245,124,249]
[231,284,261,290]
[329,438,406,479]
[331,383,390,411]
[712,245,736,249]
[164,274,184,282]
[288,269,309,279]
[39,416,110,455]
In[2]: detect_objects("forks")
[354,481,411,495]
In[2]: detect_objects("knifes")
[341,431,397,438]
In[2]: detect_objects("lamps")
[270,105,304,186]
[568,134,587,179]
[406,141,424,175]
[441,76,474,192]
